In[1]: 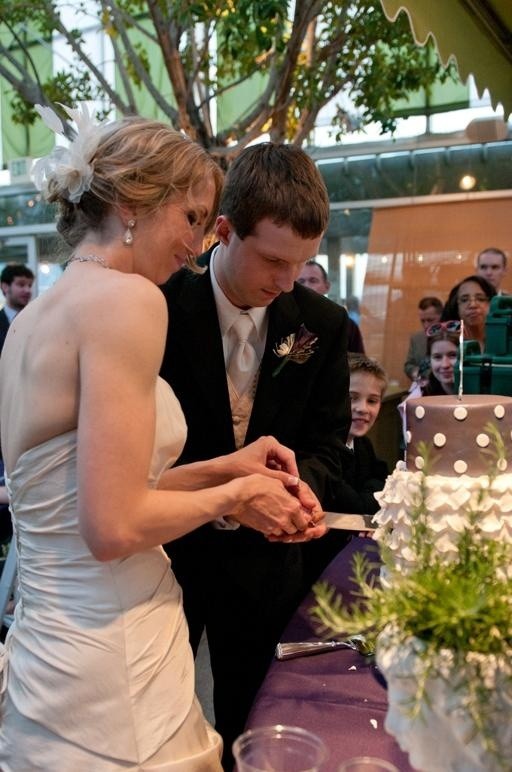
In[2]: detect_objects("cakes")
[373,394,512,772]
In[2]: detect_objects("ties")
[229,315,259,395]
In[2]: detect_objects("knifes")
[312,509,389,532]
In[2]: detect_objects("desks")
[230,535,416,771]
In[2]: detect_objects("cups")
[230,724,403,771]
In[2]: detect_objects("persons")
[0,115,314,772]
[156,144,351,771]
[0,265,34,346]
[296,260,389,558]
[403,247,506,398]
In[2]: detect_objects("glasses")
[426,321,469,338]
